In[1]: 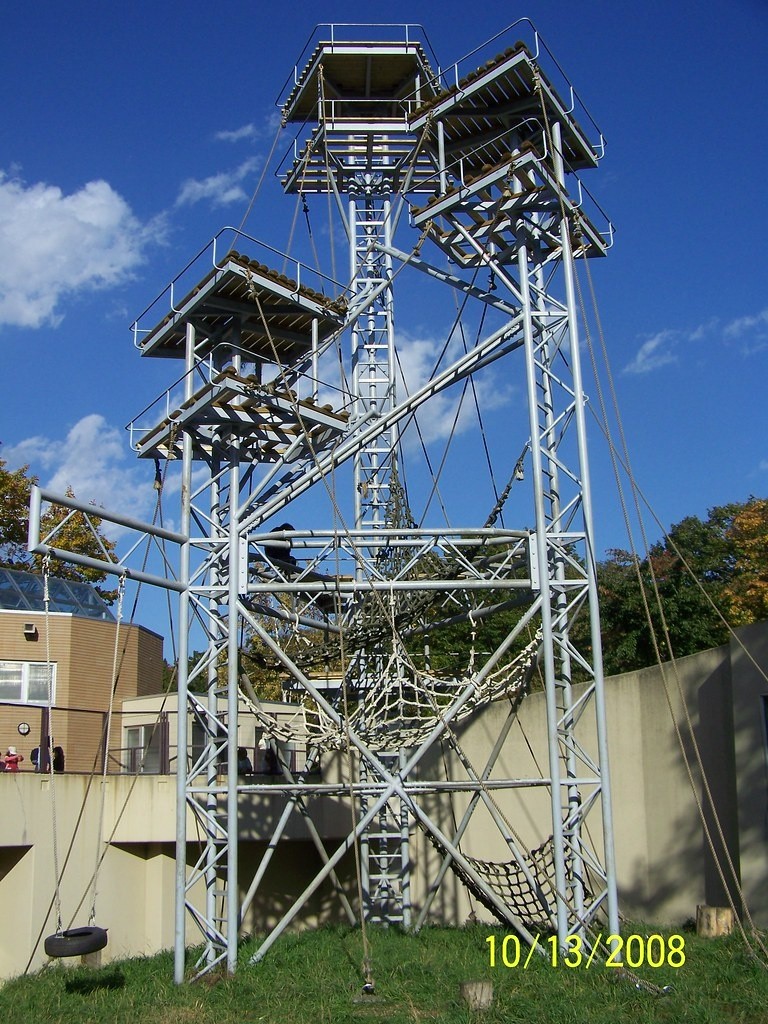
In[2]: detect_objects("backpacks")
[30,748,39,765]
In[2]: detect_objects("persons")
[264,523,297,575]
[34,735,65,774]
[4,745,23,772]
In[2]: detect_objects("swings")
[44,562,125,958]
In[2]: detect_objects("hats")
[8,746,18,754]
